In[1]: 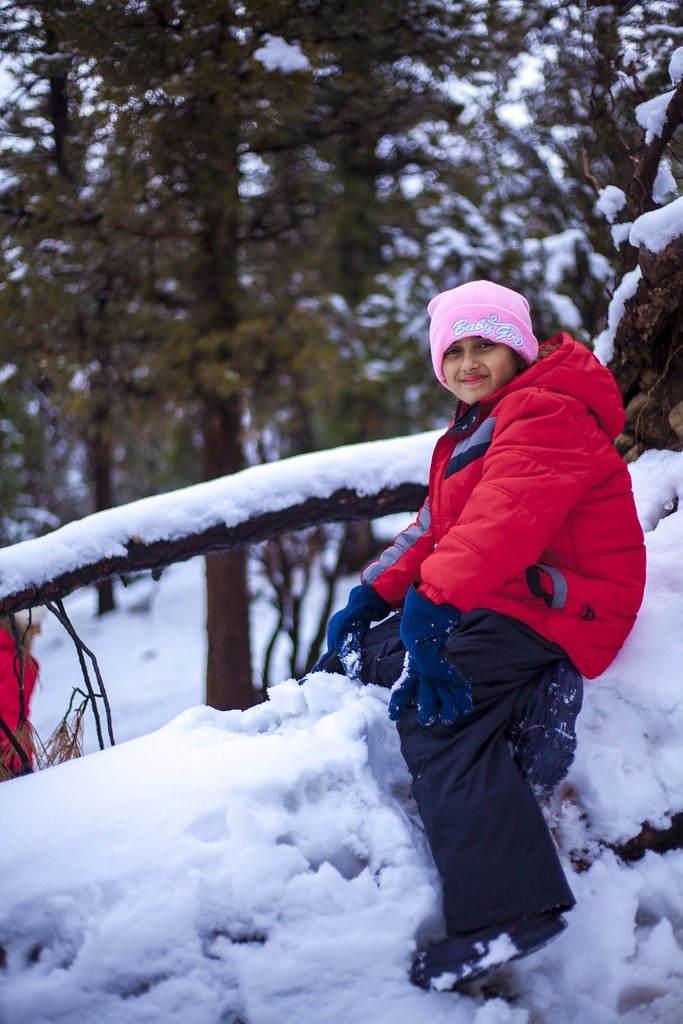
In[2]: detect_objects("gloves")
[297,585,389,687]
[388,581,475,727]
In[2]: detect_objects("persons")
[0,613,39,780]
[327,279,646,990]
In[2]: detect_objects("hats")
[427,280,538,393]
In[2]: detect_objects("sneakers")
[513,656,584,799]
[414,911,568,992]
